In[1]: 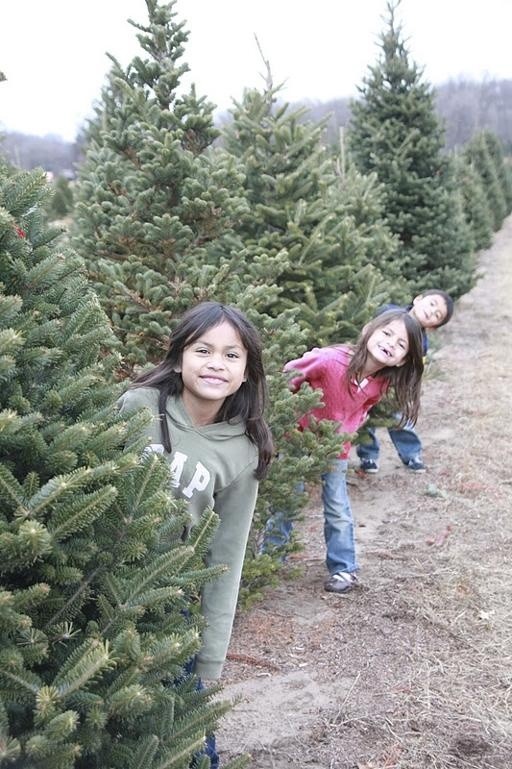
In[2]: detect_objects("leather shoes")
[325,571,356,592]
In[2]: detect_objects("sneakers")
[408,457,426,474]
[363,459,379,473]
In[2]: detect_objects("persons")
[259,309,425,594]
[350,290,454,474]
[111,302,273,769]
